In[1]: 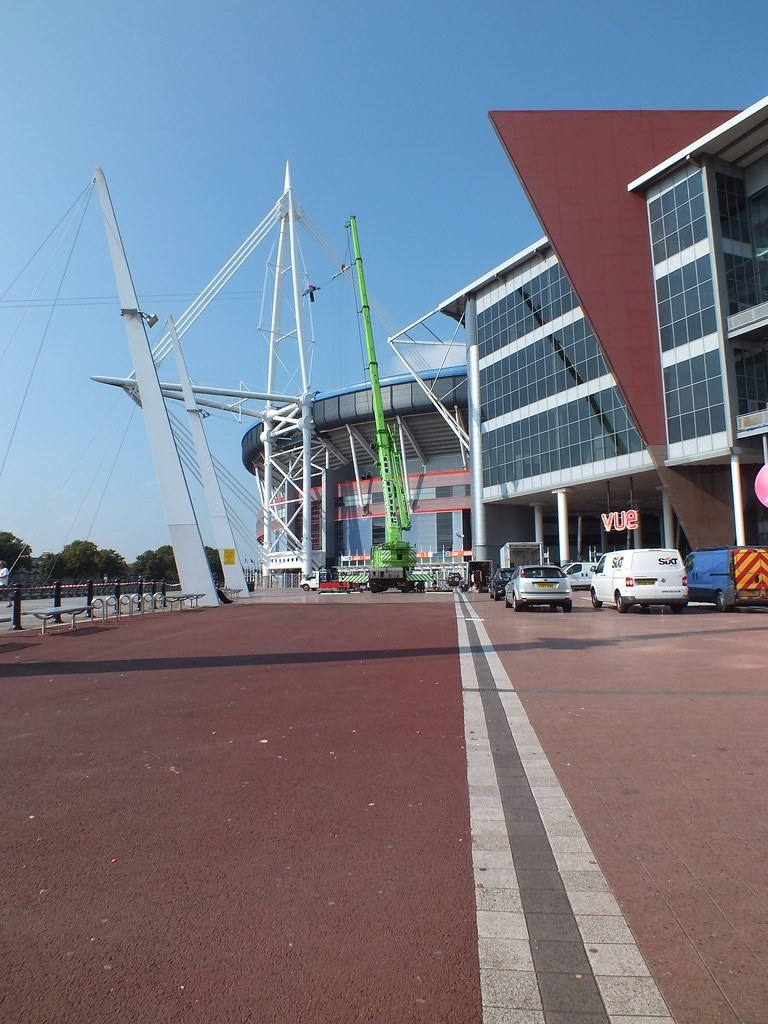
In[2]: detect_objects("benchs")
[232,589,243,598]
[33,605,95,636]
[165,596,189,611]
[182,594,206,608]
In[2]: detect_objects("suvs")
[447,572,462,586]
[487,567,518,601]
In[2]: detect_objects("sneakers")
[6,604,13,608]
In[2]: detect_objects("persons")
[0,560,13,607]
[212,570,234,604]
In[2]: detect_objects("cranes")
[336,214,438,590]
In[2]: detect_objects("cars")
[506,564,572,613]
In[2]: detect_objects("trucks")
[299,570,359,593]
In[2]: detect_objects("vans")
[681,546,768,611]
[563,562,599,591]
[590,548,689,613]
